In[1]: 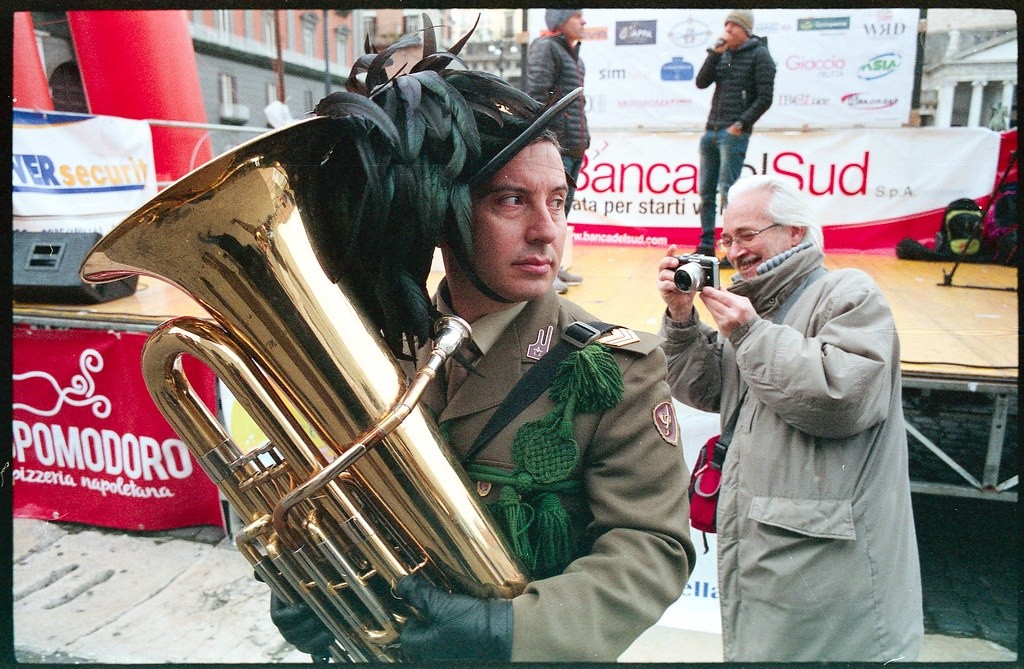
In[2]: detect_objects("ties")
[447,348,476,404]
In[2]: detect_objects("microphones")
[715,39,725,47]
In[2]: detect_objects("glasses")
[718,224,778,254]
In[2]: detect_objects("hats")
[311,13,583,359]
[725,10,754,37]
[545,8,580,32]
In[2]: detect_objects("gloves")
[270,591,332,664]
[396,578,510,668]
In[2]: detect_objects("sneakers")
[551,277,568,294]
[558,267,582,287]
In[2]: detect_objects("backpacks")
[939,199,983,263]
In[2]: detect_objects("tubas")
[74,106,526,662]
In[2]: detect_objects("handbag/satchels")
[687,435,729,534]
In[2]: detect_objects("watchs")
[735,122,743,132]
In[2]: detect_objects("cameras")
[668,251,719,294]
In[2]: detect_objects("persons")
[527,9,590,296]
[254,11,695,663]
[695,9,787,256]
[656,175,925,663]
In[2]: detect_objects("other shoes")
[720,257,734,270]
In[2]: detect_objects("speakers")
[11,230,136,303]
